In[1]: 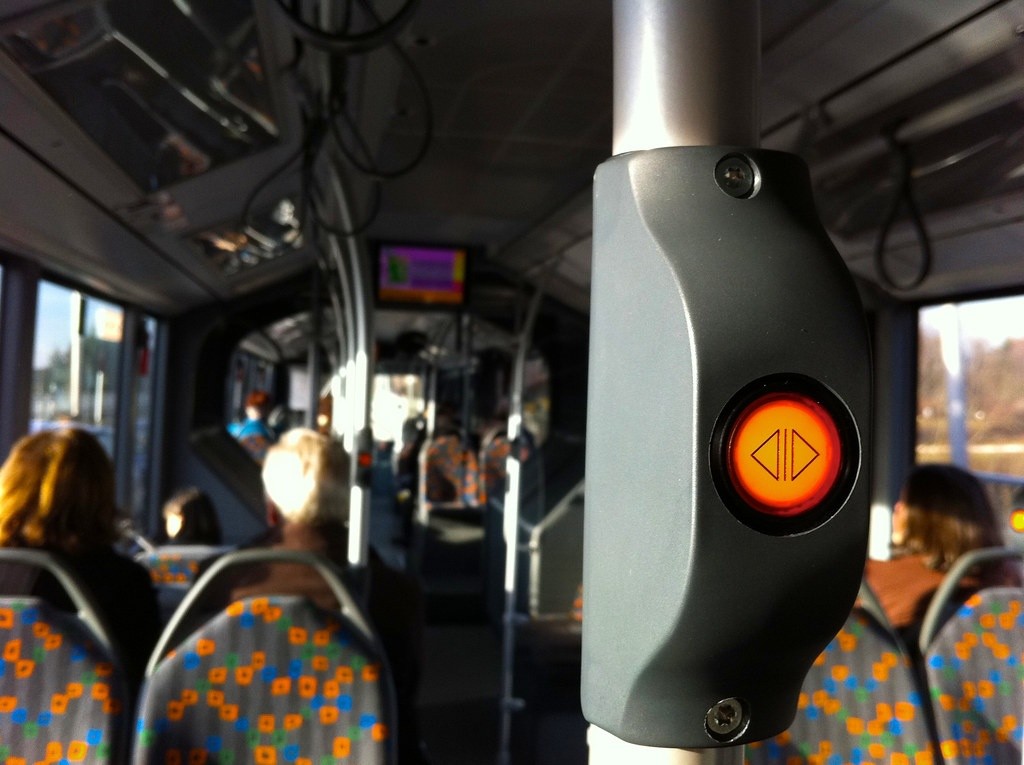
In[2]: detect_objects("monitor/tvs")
[370,238,482,313]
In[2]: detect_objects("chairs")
[0,425,1024,765]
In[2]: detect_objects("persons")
[1,388,161,702]
[226,390,277,439]
[863,462,1017,635]
[181,425,402,668]
[164,485,220,548]
